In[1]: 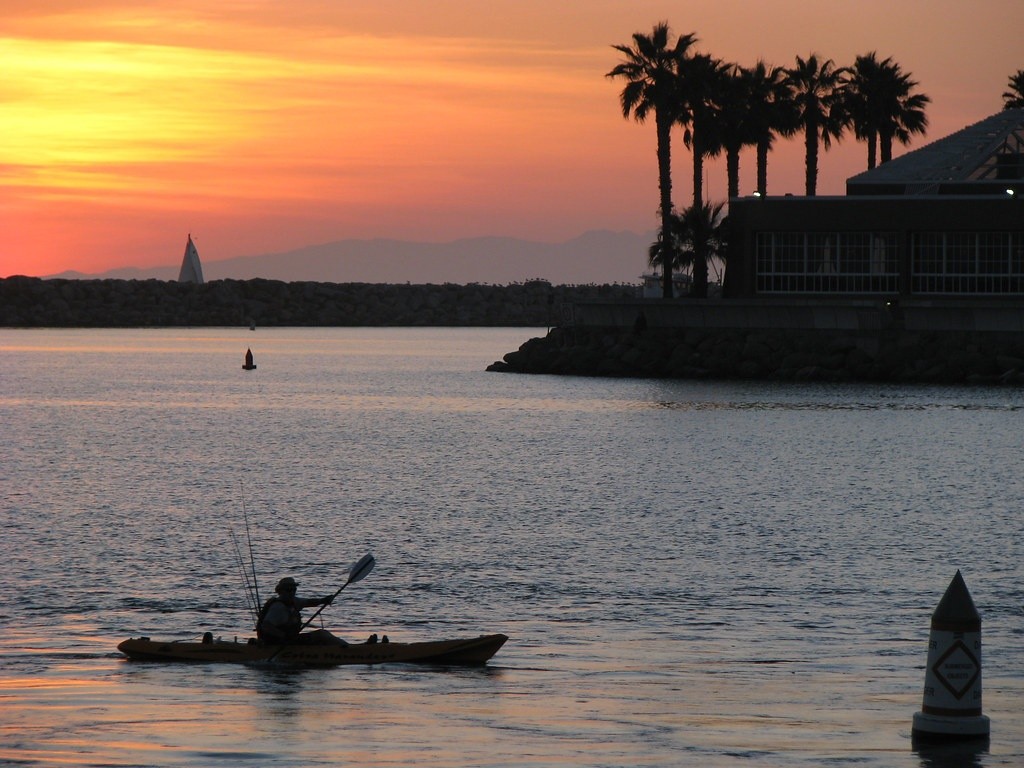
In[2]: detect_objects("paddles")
[300,552,375,632]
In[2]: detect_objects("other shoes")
[365,634,377,644]
[381,635,389,643]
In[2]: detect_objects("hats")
[275,577,300,592]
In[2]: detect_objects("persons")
[263,577,389,648]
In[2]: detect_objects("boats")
[113,479,510,667]
